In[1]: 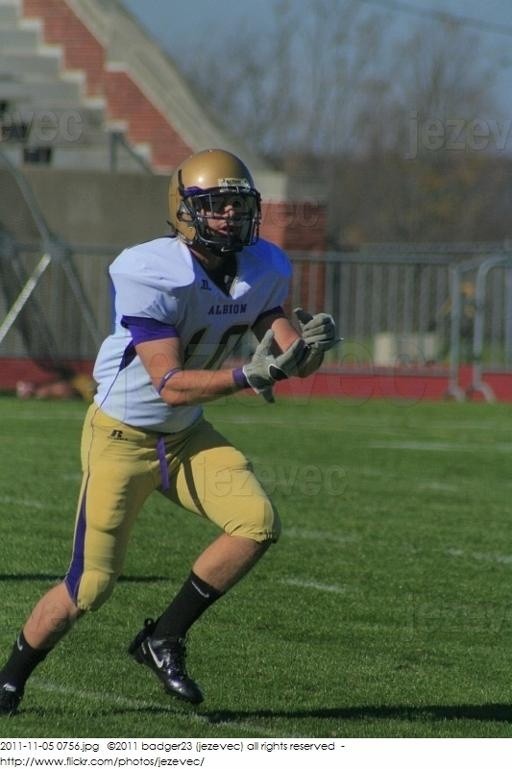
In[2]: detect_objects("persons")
[1,147,340,718]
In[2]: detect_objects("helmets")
[166,146,264,256]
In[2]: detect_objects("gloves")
[242,328,324,403]
[294,305,345,353]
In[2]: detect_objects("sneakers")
[127,616,206,706]
[0,669,26,714]
